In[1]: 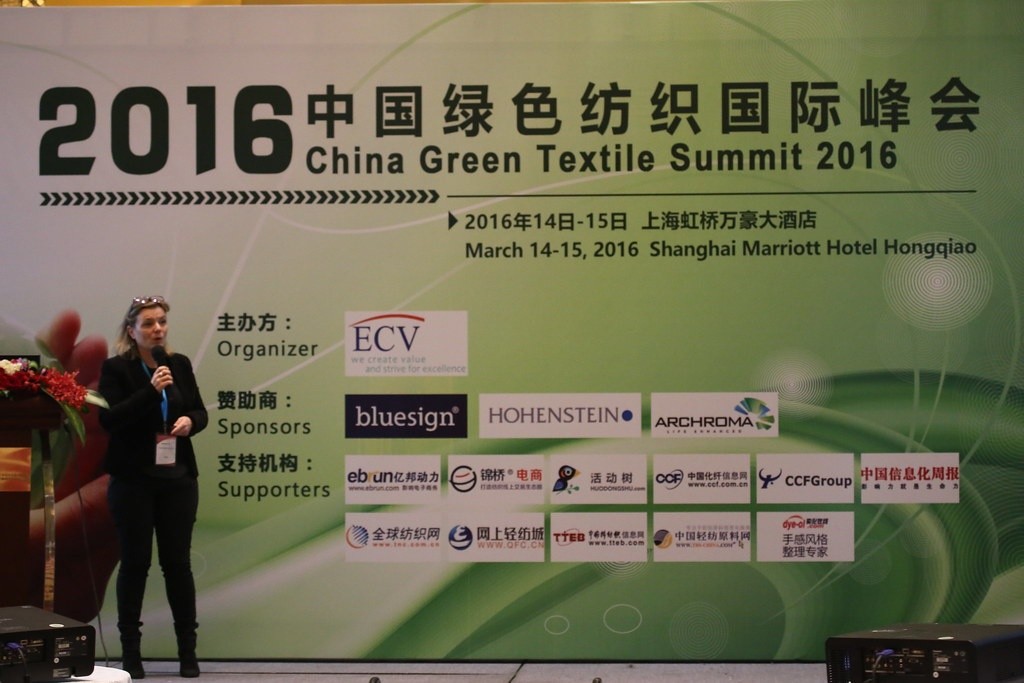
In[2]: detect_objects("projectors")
[0,606,96,683]
[825,622,1024,683]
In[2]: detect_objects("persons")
[97,295,209,679]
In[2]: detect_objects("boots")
[116,621,145,679]
[173,622,200,677]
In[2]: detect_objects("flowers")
[0,358,110,485]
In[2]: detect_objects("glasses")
[131,295,164,308]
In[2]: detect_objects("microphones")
[152,345,178,400]
